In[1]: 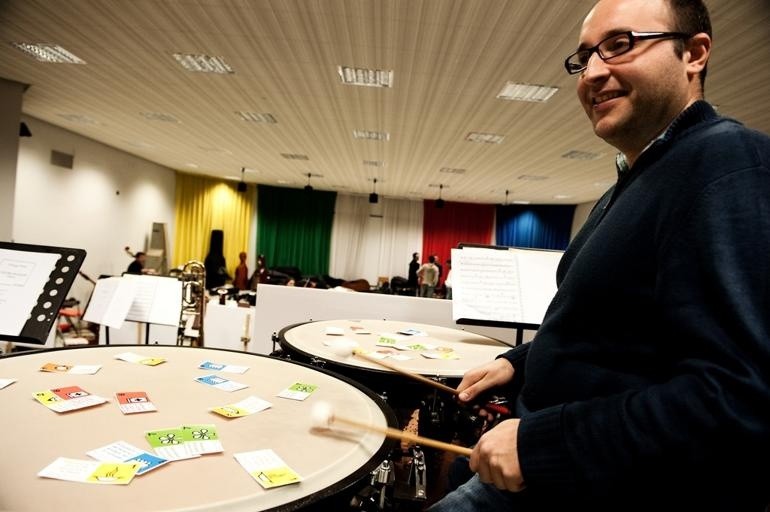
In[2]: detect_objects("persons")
[248,253,268,291]
[284,276,296,286]
[424,0,769,512]
[408,252,420,296]
[302,278,319,288]
[432,255,443,288]
[443,260,452,299]
[127,252,146,273]
[232,252,248,289]
[415,255,439,298]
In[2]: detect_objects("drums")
[270,314,517,504]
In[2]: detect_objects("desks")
[271,318,520,449]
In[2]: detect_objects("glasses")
[565,30,694,74]
